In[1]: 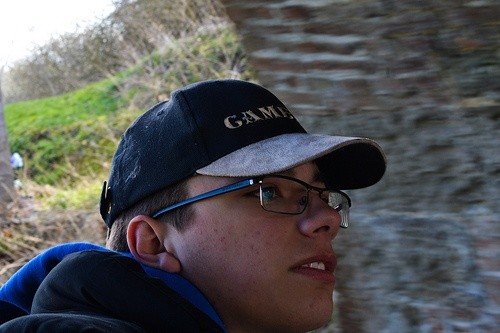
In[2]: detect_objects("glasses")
[152,174,352,228]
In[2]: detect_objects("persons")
[0,80,387,333]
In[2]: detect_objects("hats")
[99,79,386,228]
